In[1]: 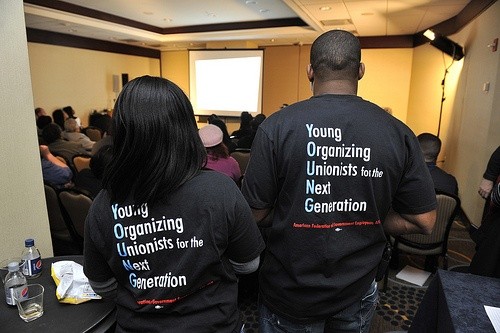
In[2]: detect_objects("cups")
[13,284,45,322]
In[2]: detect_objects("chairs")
[44,127,461,293]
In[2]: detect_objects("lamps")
[423,28,463,61]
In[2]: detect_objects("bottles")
[5,261,28,309]
[20,238,42,279]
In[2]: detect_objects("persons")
[241,29,437,333]
[35,107,113,199]
[83,76,265,333]
[474,145,500,278]
[196,112,265,178]
[417,133,458,197]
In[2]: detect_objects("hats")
[198,124,223,148]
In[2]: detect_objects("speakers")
[113,73,129,93]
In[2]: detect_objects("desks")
[408,269,500,333]
[0,256,118,333]
